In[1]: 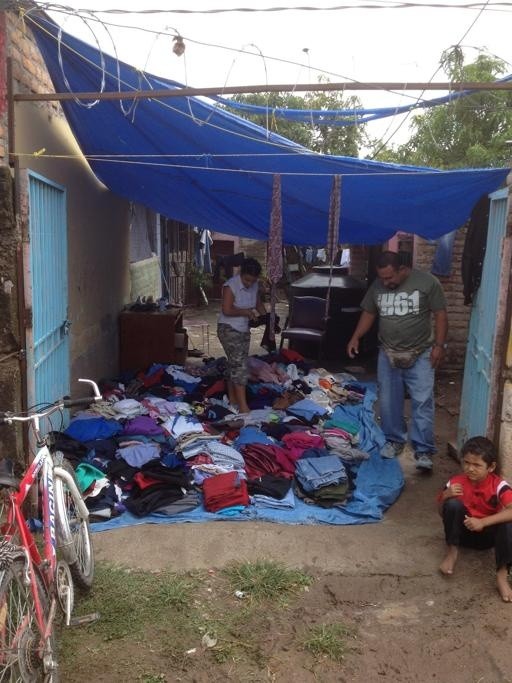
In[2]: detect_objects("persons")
[435,436,512,601]
[347,251,448,472]
[217,259,269,414]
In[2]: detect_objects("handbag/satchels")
[377,334,435,369]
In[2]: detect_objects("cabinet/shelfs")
[119,302,190,378]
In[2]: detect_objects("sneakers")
[416,452,433,471]
[380,440,404,459]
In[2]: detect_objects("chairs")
[278,295,329,369]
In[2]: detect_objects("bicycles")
[0,377,104,683]
